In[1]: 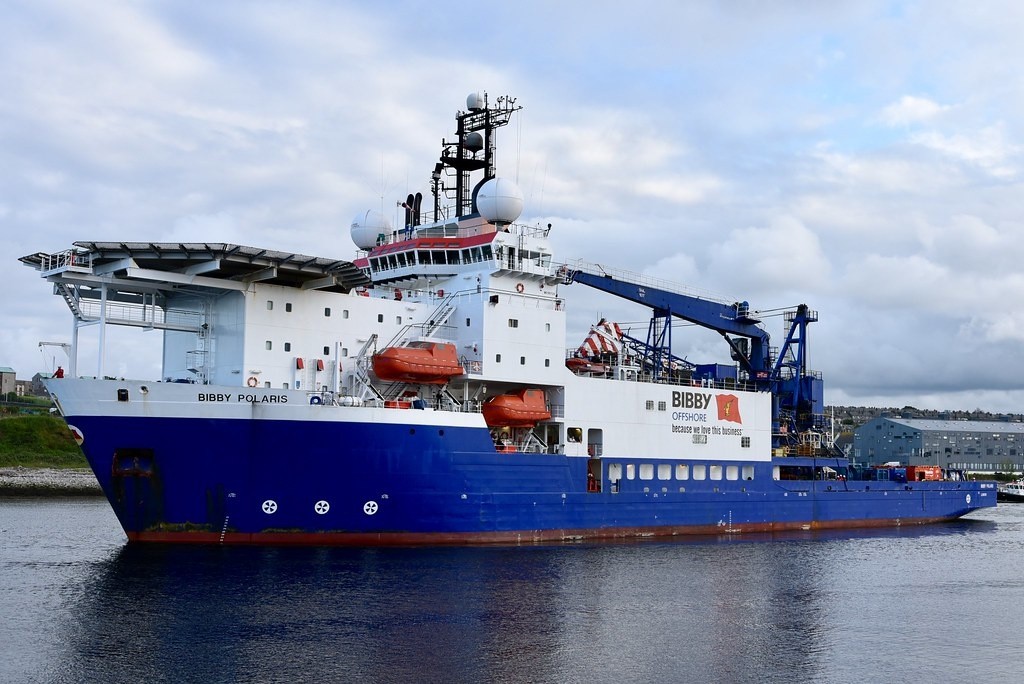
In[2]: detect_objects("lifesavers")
[247,377,258,387]
[517,283,524,293]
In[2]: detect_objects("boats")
[17,89,998,547]
[997,478,1024,502]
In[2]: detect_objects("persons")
[837,474,845,481]
[436,390,443,409]
[52,365,64,378]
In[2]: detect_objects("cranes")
[36,342,72,375]
